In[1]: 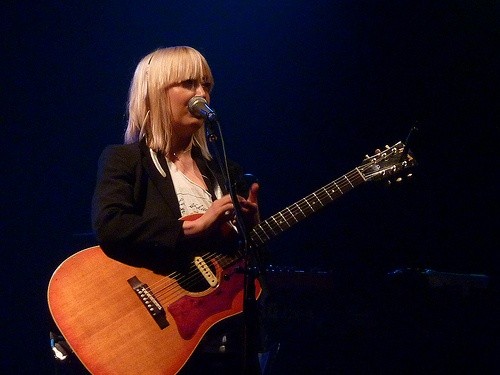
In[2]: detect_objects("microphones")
[188,97,217,121]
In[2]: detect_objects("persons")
[91,47,262,375]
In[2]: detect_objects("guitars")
[45,140,417,375]
